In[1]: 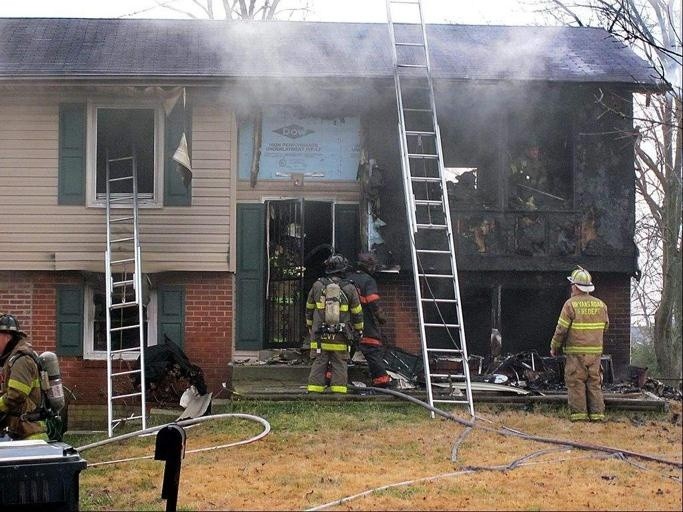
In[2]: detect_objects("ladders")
[105,142,147,437]
[386,0,476,421]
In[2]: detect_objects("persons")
[305,255,364,396]
[268,223,306,346]
[550,268,610,422]
[1,314,51,448]
[348,253,400,391]
[511,141,549,246]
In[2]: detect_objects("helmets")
[0,313,27,336]
[283,223,306,239]
[352,254,376,274]
[324,252,348,274]
[568,267,595,292]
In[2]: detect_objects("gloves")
[355,330,363,340]
